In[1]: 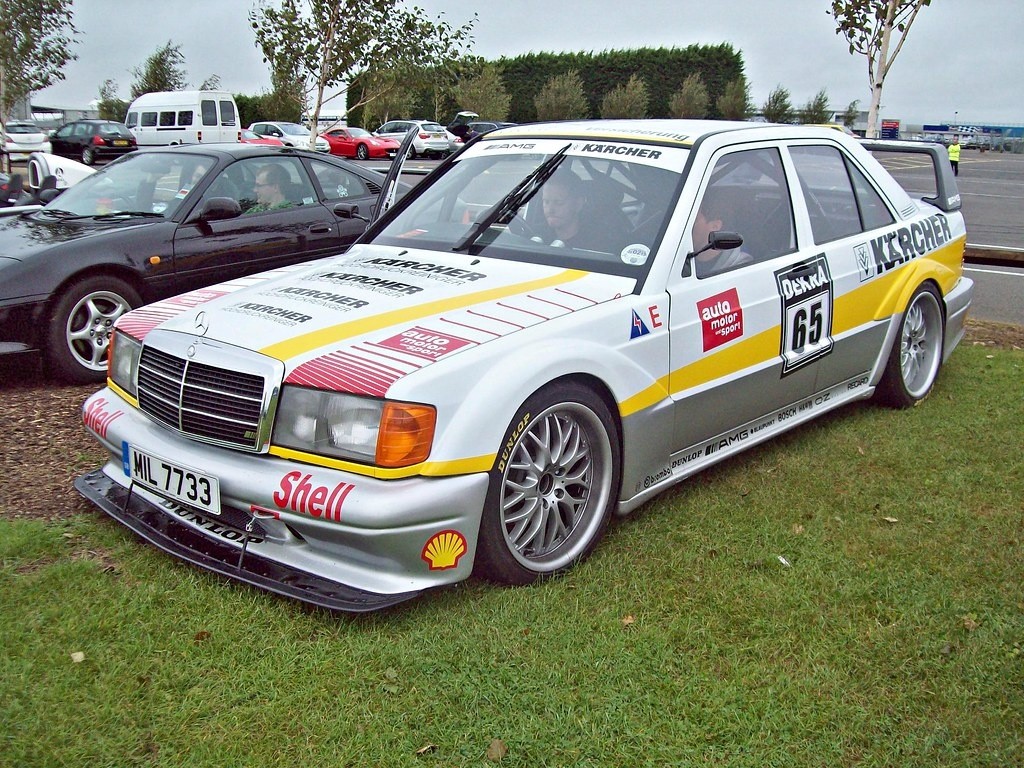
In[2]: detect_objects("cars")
[440,111,480,159]
[1,121,52,162]
[49,119,138,165]
[922,133,1021,154]
[248,122,330,154]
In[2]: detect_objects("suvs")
[468,120,517,136]
[374,117,449,159]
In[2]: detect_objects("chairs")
[583,181,627,250]
[694,184,769,265]
[203,175,245,213]
[286,183,311,205]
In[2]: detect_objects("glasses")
[256,183,274,189]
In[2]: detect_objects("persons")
[469,128,479,139]
[948,137,960,176]
[529,168,611,253]
[136,164,228,219]
[239,164,300,216]
[691,196,754,275]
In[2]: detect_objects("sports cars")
[319,127,402,159]
[240,129,285,154]
[75,119,976,610]
[0,141,474,380]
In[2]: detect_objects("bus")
[125,90,242,163]
